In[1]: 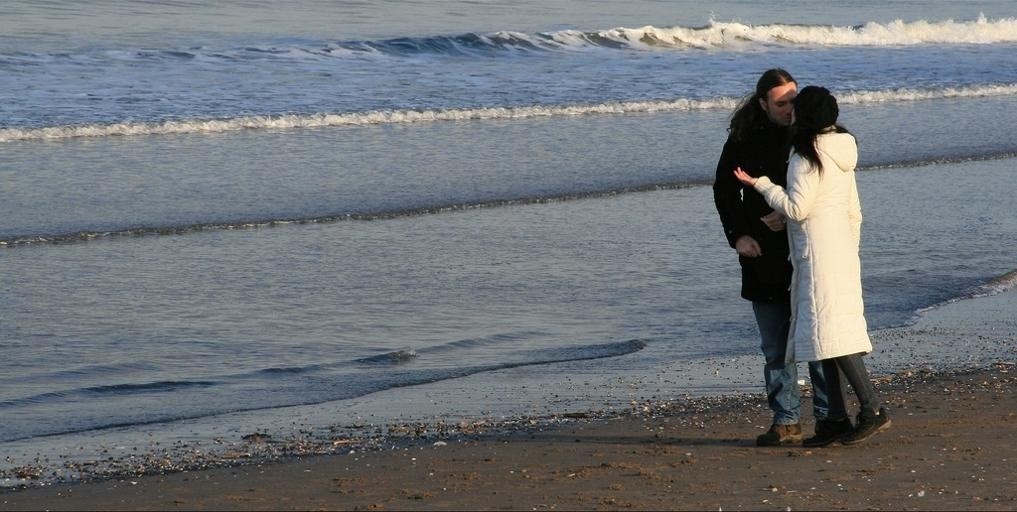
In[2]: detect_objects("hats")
[790,85,840,128]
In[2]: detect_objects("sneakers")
[755,417,804,446]
[841,404,893,445]
[803,415,856,446]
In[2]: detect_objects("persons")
[713,69,829,446]
[731,83,895,448]
